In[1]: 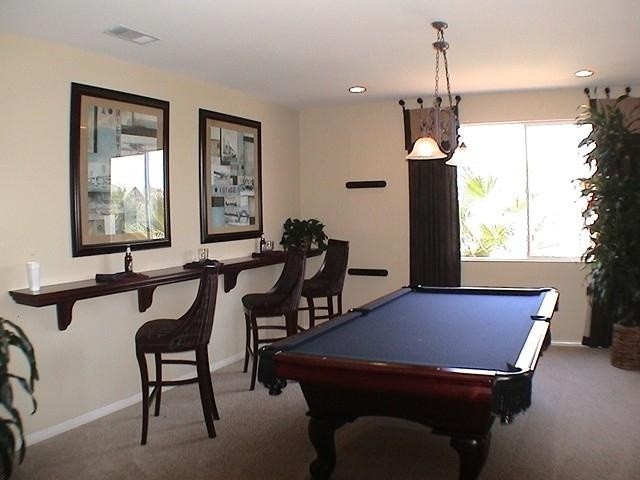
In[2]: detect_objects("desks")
[257,282,562,478]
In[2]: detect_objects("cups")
[267,241,272,251]
[198,249,208,262]
[26,260,40,290]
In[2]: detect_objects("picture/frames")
[71,81,264,258]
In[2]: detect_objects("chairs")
[134,266,216,445]
[297,240,348,334]
[241,243,308,392]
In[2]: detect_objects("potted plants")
[571,86,638,371]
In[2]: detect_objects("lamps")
[407,20,479,166]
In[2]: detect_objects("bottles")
[124,244,133,274]
[260,233,266,253]
